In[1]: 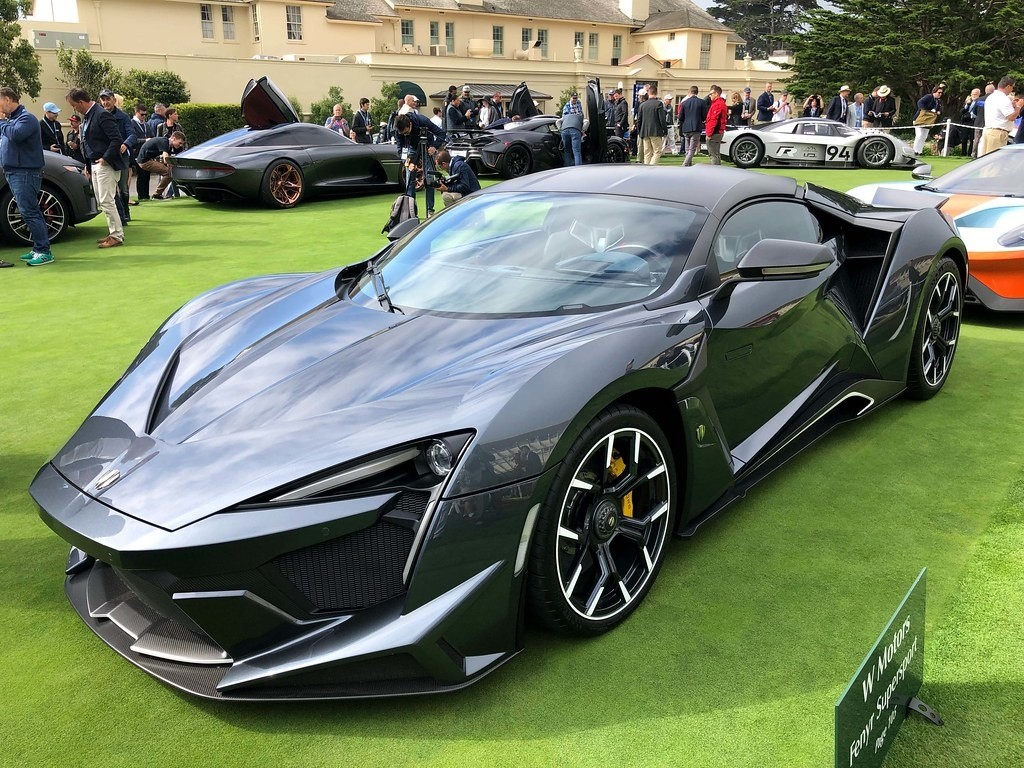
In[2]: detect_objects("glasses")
[708,92,716,98]
[1010,84,1016,89]
[463,91,471,94]
[137,111,148,117]
[400,127,410,136]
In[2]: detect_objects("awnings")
[394,81,427,106]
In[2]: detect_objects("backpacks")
[381,194,418,234]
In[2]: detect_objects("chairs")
[715,207,769,271]
[544,201,642,263]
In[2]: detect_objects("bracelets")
[1017,106,1021,109]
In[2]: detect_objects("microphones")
[427,170,441,176]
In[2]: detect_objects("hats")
[412,95,419,102]
[43,102,63,113]
[570,91,578,97]
[635,88,647,96]
[744,87,751,93]
[838,86,851,94]
[609,89,614,96]
[478,96,494,107]
[665,95,673,101]
[67,114,81,122]
[99,89,114,98]
[782,90,788,96]
[877,84,891,97]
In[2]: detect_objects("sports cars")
[700,117,928,169]
[28,163,968,705]
[843,143,1024,312]
[0,150,102,247]
[165,75,424,209]
[443,76,632,180]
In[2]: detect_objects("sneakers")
[19,250,54,265]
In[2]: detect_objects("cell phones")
[335,116,342,120]
[54,144,62,148]
[787,103,789,105]
[812,96,818,99]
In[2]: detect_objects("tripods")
[404,127,448,218]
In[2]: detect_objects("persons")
[757,82,792,123]
[826,85,896,133]
[727,87,757,126]
[395,112,447,217]
[430,85,503,141]
[376,95,420,143]
[803,94,825,117]
[563,92,583,117]
[678,84,728,166]
[971,85,994,157]
[66,88,127,248]
[913,86,943,156]
[0,86,54,266]
[100,89,188,226]
[960,88,981,157]
[978,76,1024,157]
[325,98,375,144]
[40,102,84,164]
[634,84,683,165]
[604,88,628,139]
[556,114,589,167]
[434,151,481,207]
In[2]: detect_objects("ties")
[842,97,845,119]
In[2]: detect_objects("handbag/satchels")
[914,109,937,129]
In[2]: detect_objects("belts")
[988,128,1009,133]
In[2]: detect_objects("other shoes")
[122,193,180,226]
[428,210,434,218]
[674,154,683,157]
[97,234,126,248]
[0,259,14,267]
